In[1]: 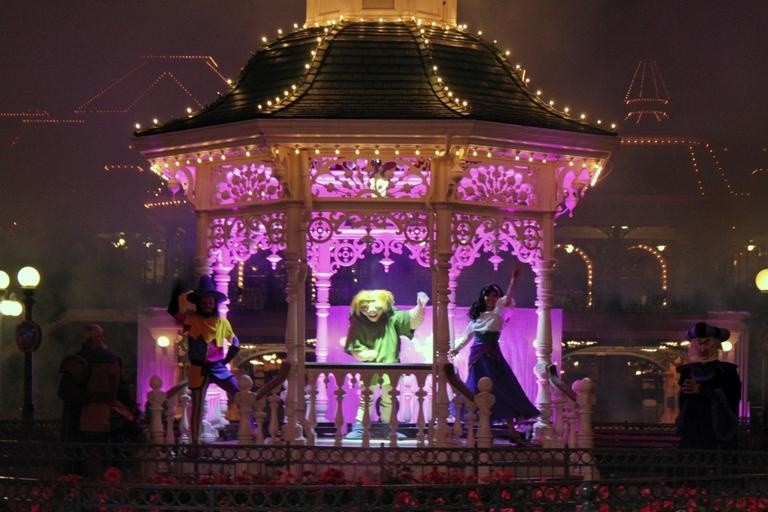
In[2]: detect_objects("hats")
[186,275,228,304]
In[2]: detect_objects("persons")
[450,264,541,443]
[60,323,145,476]
[166,274,239,441]
[342,289,429,439]
[676,321,743,449]
